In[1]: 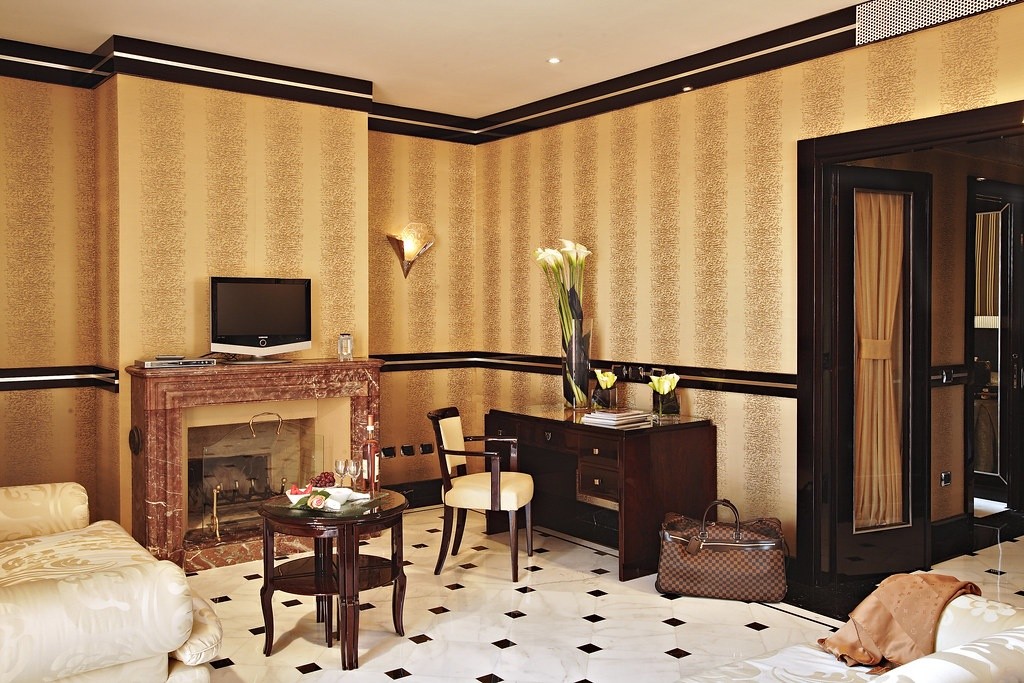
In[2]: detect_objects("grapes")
[309,472,335,487]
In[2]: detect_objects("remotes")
[155,354,185,360]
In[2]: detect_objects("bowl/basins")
[286,487,352,505]
[305,483,339,489]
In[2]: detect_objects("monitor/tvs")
[209,276,312,364]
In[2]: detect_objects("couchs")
[0,479,224,683]
[672,572,1024,682]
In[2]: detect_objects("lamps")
[387,222,436,279]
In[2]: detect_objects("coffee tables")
[257,484,409,670]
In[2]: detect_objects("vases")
[652,386,685,414]
[591,386,618,408]
[563,317,595,410]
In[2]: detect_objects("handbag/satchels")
[655,500,788,602]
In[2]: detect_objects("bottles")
[337,334,353,362]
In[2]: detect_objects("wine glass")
[347,461,361,491]
[335,458,347,487]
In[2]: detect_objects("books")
[581,408,651,430]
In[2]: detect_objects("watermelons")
[290,483,312,494]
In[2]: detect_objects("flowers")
[535,238,589,323]
[594,369,620,389]
[647,373,680,392]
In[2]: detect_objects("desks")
[483,407,717,581]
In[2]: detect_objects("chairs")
[426,407,533,584]
